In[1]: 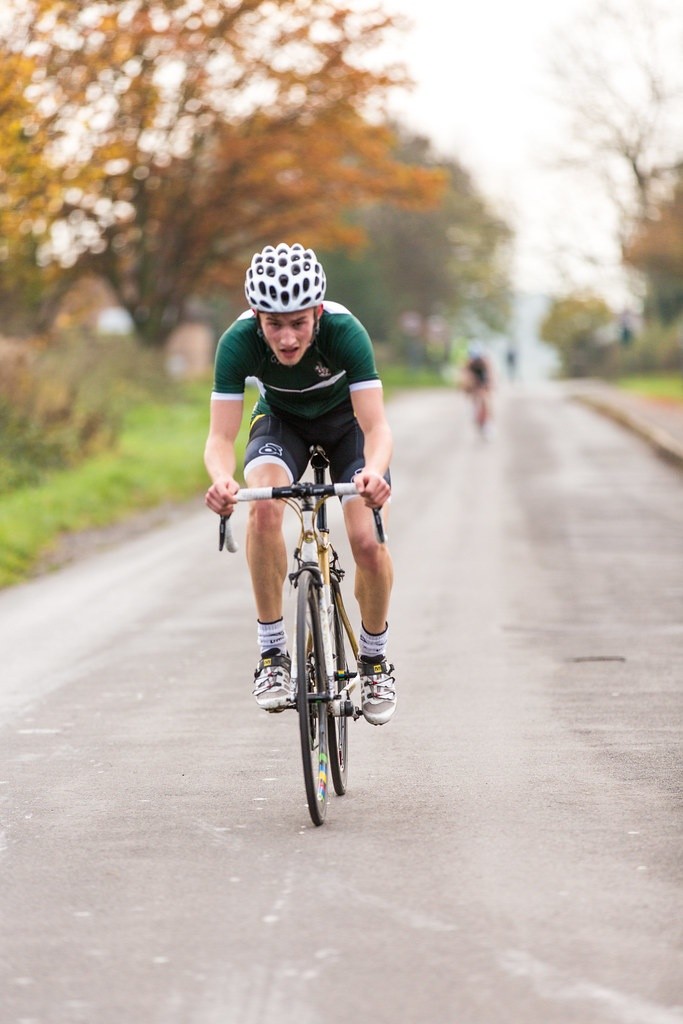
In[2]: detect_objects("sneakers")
[252,647,292,710]
[357,653,396,726]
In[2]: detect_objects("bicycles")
[216,443,368,828]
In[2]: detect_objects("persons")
[464,344,490,424]
[203,244,398,725]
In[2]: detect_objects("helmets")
[245,241,327,315]
